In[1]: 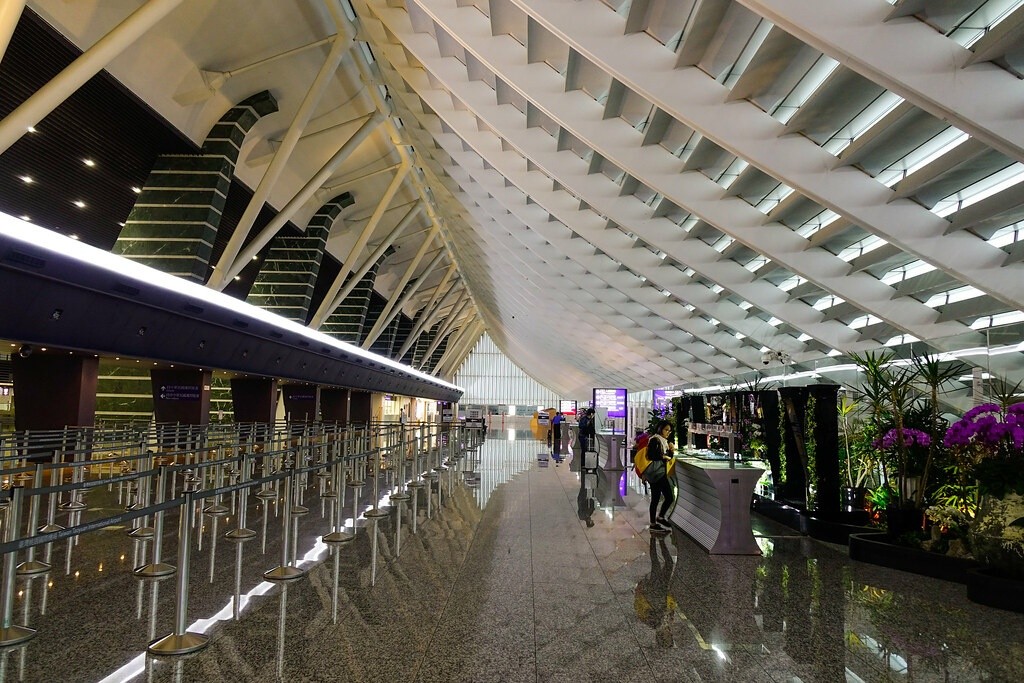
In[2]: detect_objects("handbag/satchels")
[666,457,675,478]
[587,420,595,438]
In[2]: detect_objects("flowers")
[872,403,1024,505]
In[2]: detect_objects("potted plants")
[837,344,1024,613]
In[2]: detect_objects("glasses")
[662,428,672,434]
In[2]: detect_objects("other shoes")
[581,465,586,469]
[649,530,657,538]
[655,529,671,539]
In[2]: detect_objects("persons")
[400,408,406,431]
[634,420,674,532]
[578,408,595,468]
[552,412,564,439]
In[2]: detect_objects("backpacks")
[631,432,665,494]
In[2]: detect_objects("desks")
[668,457,766,554]
[569,449,581,472]
[596,471,627,507]
[561,438,570,454]
[561,422,626,471]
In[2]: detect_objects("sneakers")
[655,518,672,530]
[649,523,666,534]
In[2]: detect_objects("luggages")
[584,434,598,473]
[548,421,552,440]
